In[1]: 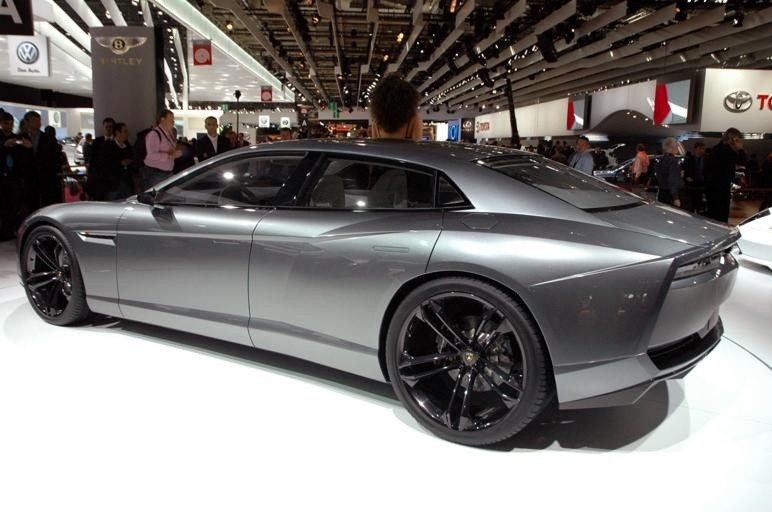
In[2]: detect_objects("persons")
[446,136,600,177]
[259,124,334,144]
[367,71,431,198]
[633,125,772,225]
[0,107,252,243]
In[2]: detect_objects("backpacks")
[134,127,175,160]
